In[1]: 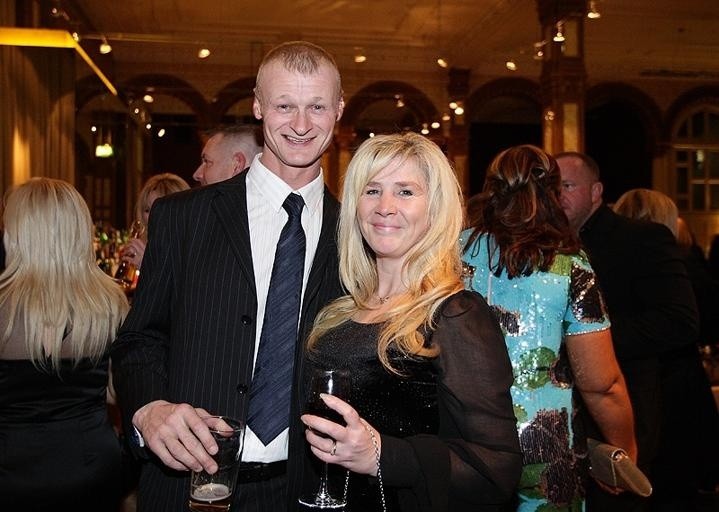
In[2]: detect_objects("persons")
[454,140,640,512]
[2,176,142,511]
[545,150,718,512]
[188,123,263,189]
[120,172,191,273]
[285,127,523,512]
[612,189,679,242]
[109,39,356,510]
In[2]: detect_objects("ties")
[245,192,306,445]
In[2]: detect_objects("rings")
[330,441,336,455]
[130,251,138,257]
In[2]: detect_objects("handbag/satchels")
[588,437,652,499]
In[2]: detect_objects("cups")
[186,416,246,512]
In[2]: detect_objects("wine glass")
[298,364,352,509]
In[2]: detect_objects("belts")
[237,461,285,484]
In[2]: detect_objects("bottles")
[95,220,145,288]
